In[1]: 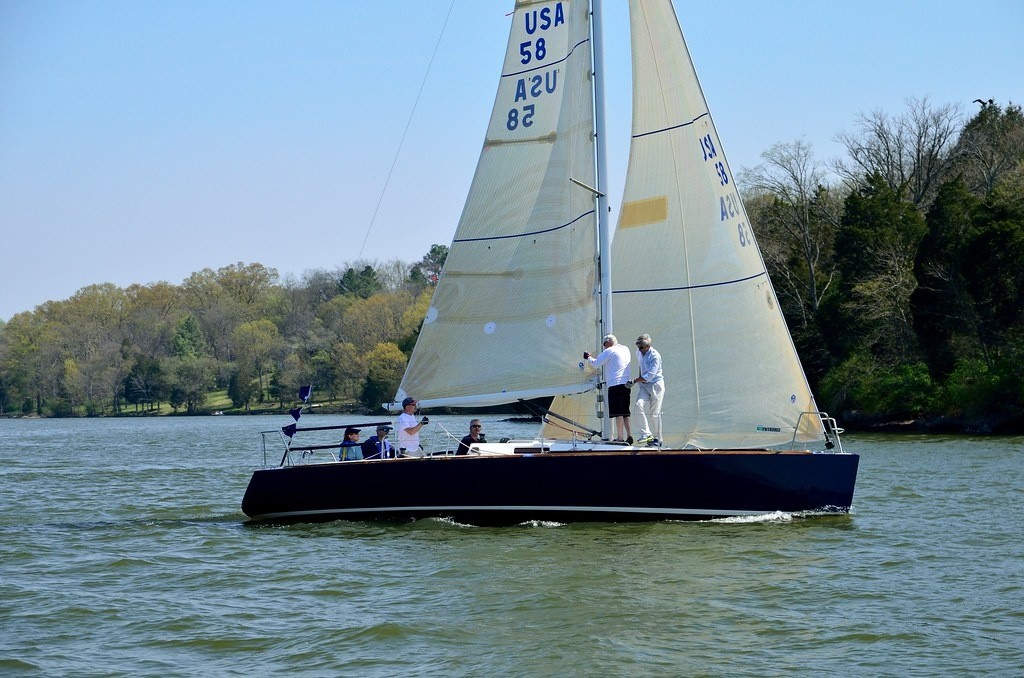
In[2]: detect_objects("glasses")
[470,425,481,428]
[407,404,415,406]
[603,340,610,346]
[380,428,389,432]
[638,346,644,349]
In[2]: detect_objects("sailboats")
[242,0,860,528]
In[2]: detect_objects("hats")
[377,425,393,430]
[345,429,361,436]
[402,397,419,407]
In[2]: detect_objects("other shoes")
[625,436,633,445]
[637,435,654,444]
[648,439,662,446]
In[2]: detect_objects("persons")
[397,397,429,456]
[456,419,487,455]
[583,334,634,446]
[338,425,398,460]
[624,334,665,446]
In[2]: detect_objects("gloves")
[625,379,636,389]
[420,416,429,425]
[583,351,590,360]
